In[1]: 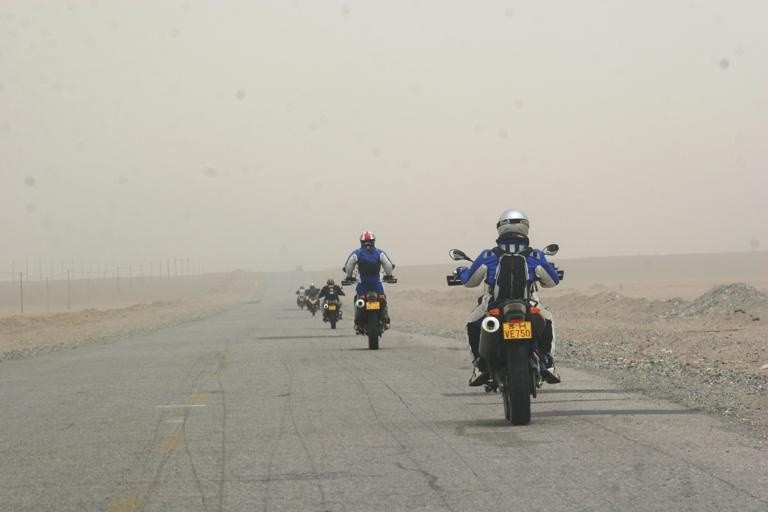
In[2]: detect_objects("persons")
[318,280,344,309]
[344,231,394,324]
[456,208,561,386]
[305,283,321,308]
[296,286,306,304]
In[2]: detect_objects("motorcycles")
[342,264,397,349]
[295,292,306,310]
[305,289,321,315]
[446,243,565,426]
[317,288,344,329]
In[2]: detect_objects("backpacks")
[492,247,533,303]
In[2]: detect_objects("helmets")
[360,232,375,246]
[497,209,529,244]
[328,279,334,285]
[310,284,315,288]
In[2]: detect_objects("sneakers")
[469,356,489,386]
[541,365,560,383]
[354,324,363,330]
[384,319,390,324]
